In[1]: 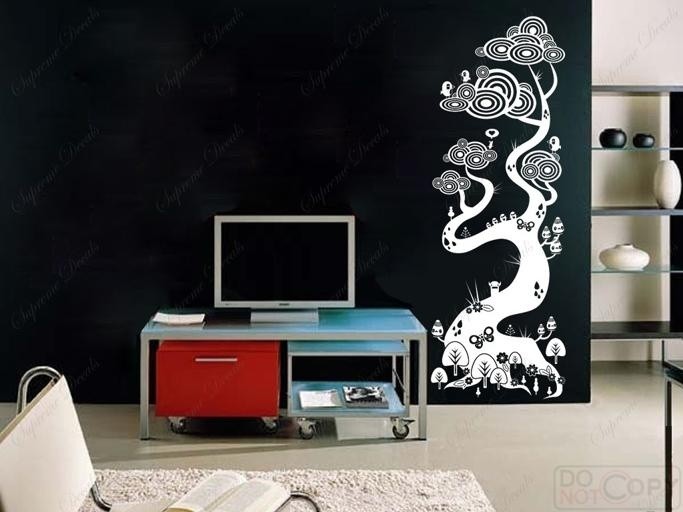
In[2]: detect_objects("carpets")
[80,467,495,511]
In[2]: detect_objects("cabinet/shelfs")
[591,85,683,340]
[142,310,428,441]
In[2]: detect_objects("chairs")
[0,367,324,512]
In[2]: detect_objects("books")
[164,469,291,512]
[298,388,341,409]
[342,385,389,408]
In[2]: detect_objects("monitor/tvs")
[214,215,355,323]
